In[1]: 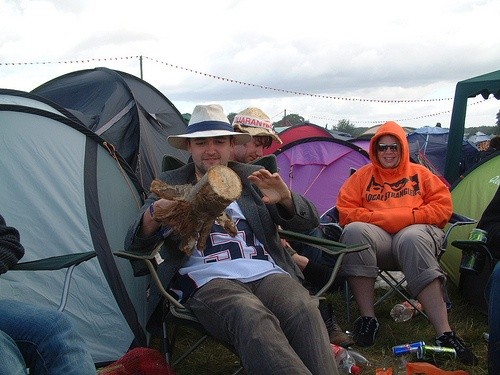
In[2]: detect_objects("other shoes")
[325,317,350,346]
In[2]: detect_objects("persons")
[336,121,479,366]
[125,104,340,375]
[0,298,98,375]
[228,107,350,347]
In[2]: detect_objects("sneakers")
[353,317,379,349]
[435,332,478,365]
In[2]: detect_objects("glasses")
[376,143,400,153]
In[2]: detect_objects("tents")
[29,67,191,191]
[436,151,500,311]
[445,70,500,184]
[0,88,180,370]
[405,126,480,176]
[262,123,375,218]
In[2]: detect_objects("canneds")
[392,341,425,356]
[417,345,457,361]
[461,229,488,272]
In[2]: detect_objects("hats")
[166,104,253,151]
[231,107,283,145]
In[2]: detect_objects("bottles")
[390,298,424,322]
[329,342,361,375]
[346,347,370,367]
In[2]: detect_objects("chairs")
[113,154,477,375]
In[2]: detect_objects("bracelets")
[150,202,161,223]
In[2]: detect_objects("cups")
[375,367,392,375]
